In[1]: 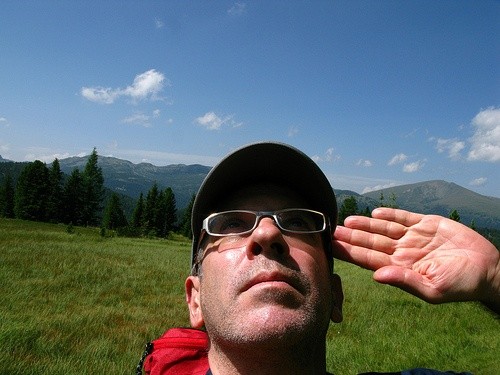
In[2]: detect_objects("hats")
[191,142,338,270]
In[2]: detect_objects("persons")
[137,139,499,375]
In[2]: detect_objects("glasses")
[196,208,334,265]
